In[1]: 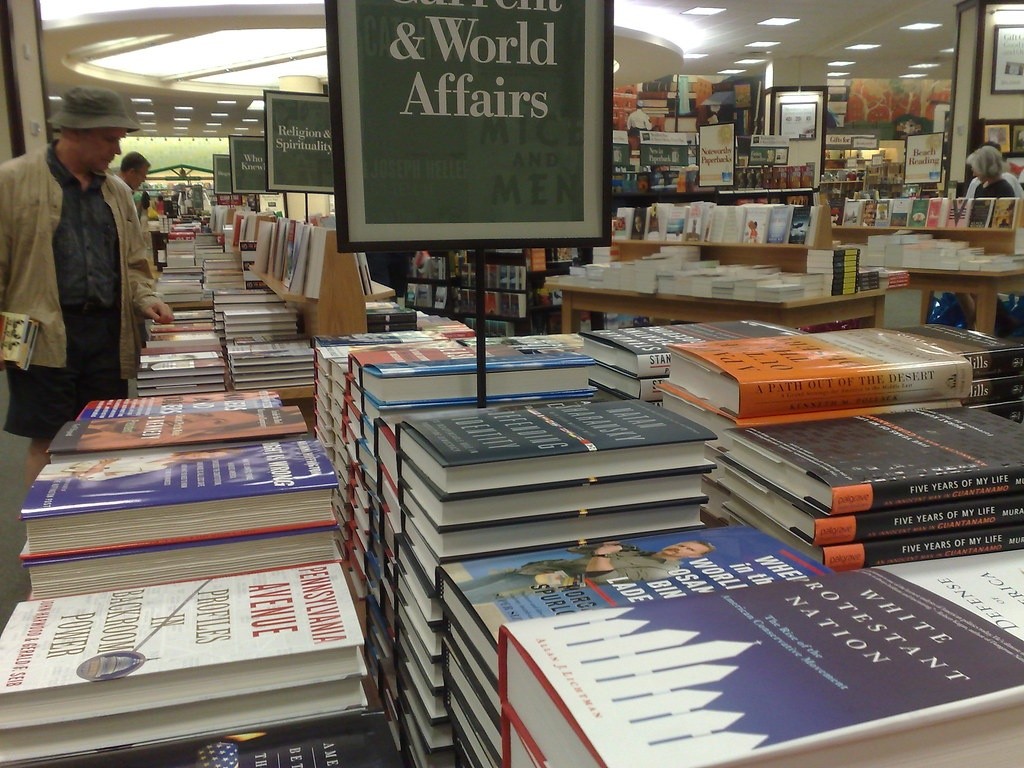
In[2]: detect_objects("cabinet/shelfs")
[403,76,1021,337]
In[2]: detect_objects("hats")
[47,87,140,133]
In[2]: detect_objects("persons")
[0,86,173,441]
[572,165,1024,304]
[626,104,653,134]
[965,140,1024,198]
[410,249,526,338]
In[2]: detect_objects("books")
[577,319,1024,568]
[0,390,403,768]
[400,525,1024,768]
[136,204,374,397]
[0,311,40,371]
[311,320,718,562]
